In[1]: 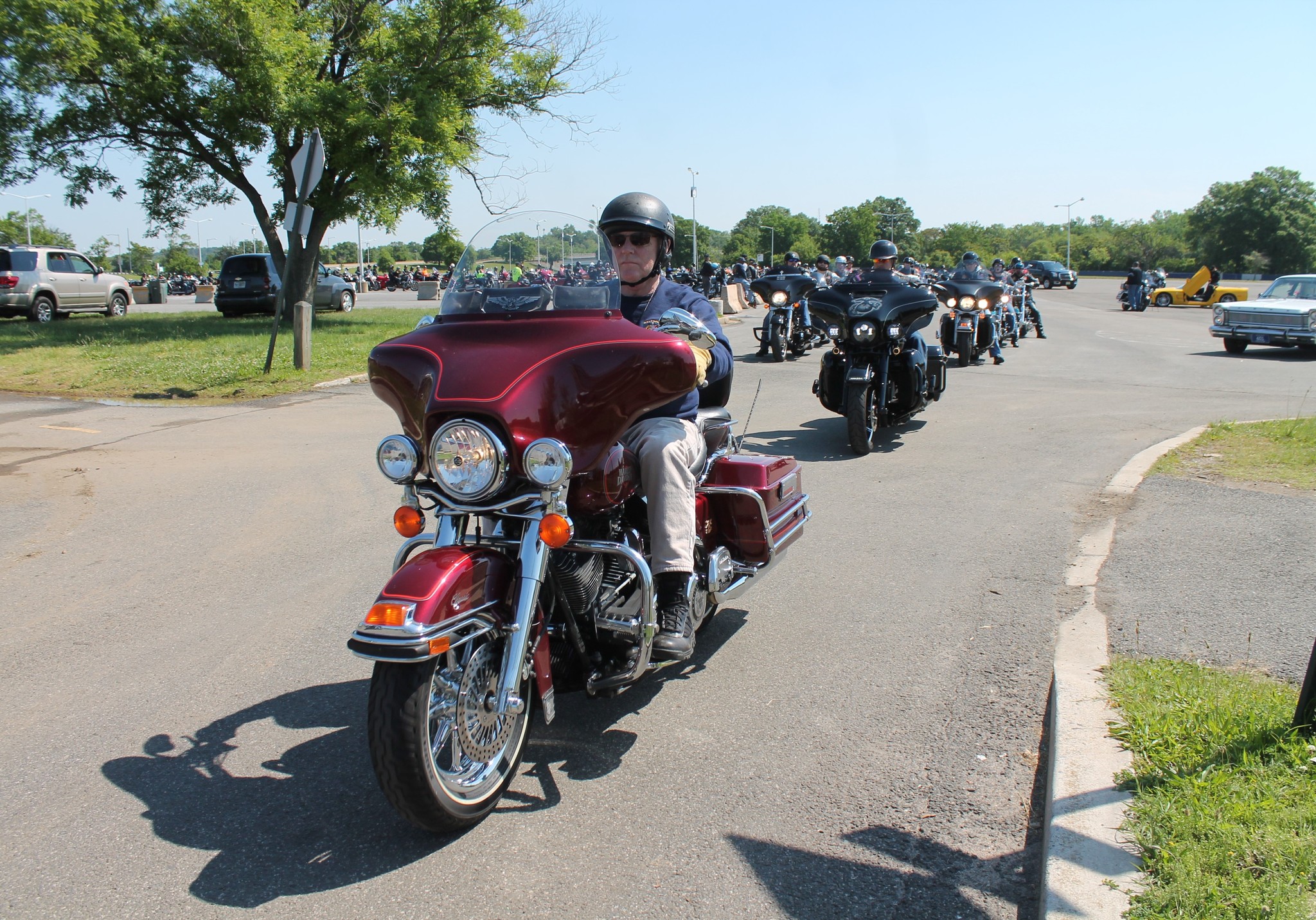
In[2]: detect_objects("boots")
[651,571,695,659]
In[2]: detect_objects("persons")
[1126,261,1142,311]
[689,262,696,273]
[665,262,675,280]
[868,240,928,397]
[755,251,854,356]
[1201,265,1221,307]
[483,192,732,660]
[141,271,217,295]
[700,254,716,300]
[952,252,1046,364]
[733,254,768,308]
[332,260,612,292]
[897,257,947,275]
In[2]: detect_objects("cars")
[1209,273,1316,354]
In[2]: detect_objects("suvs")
[0,243,133,325]
[1021,260,1078,290]
[214,252,357,319]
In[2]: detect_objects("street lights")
[505,238,513,271]
[684,235,698,270]
[760,225,774,268]
[1054,197,1085,269]
[107,233,122,273]
[546,246,554,268]
[557,226,569,264]
[871,212,914,242]
[328,237,336,264]
[242,222,260,254]
[414,250,418,261]
[207,238,217,267]
[566,234,577,270]
[688,167,700,270]
[0,191,52,245]
[361,239,376,264]
[592,204,602,261]
[183,218,213,262]
[529,217,547,264]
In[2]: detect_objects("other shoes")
[1014,342,1019,347]
[756,348,768,356]
[1036,334,1048,339]
[805,328,811,337]
[993,357,1004,364]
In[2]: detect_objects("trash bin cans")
[149,280,168,304]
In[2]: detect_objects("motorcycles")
[1116,266,1169,312]
[346,210,815,835]
[334,260,1037,458]
[127,274,219,295]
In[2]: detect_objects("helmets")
[140,269,216,281]
[692,262,697,267]
[596,192,675,253]
[991,255,1024,270]
[783,251,800,260]
[962,251,979,263]
[335,261,612,279]
[904,256,929,269]
[817,254,830,264]
[835,255,854,264]
[869,239,898,259]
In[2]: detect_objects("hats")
[737,253,758,267]
[705,253,710,258]
[1210,265,1216,270]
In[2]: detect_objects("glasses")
[994,264,1003,267]
[788,259,799,262]
[608,232,661,247]
[965,262,975,266]
[818,260,828,264]
[872,259,891,263]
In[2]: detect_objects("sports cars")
[1149,265,1249,308]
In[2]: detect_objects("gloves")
[685,341,713,390]
